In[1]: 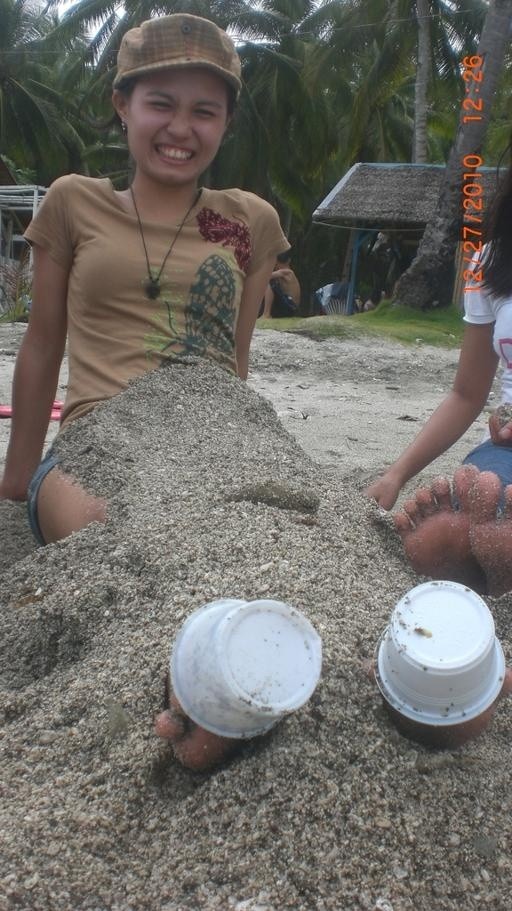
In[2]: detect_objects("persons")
[253,249,303,321]
[359,135,508,614]
[1,8,510,774]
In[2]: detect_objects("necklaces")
[126,180,203,300]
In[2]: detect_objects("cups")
[167,594,324,740]
[371,581,506,727]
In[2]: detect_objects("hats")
[112,12,242,101]
